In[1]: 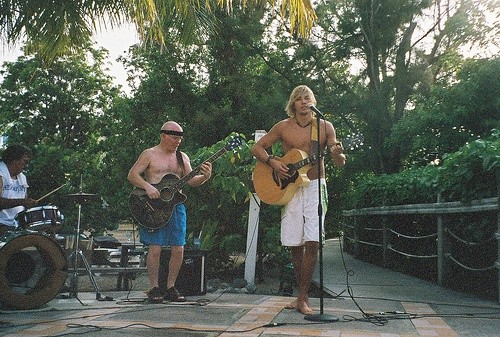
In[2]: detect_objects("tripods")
[67,204,103,300]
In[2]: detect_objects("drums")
[14,205,64,230]
[0,231,68,310]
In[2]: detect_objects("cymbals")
[68,193,96,196]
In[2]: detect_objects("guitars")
[127,131,242,229]
[252,131,364,206]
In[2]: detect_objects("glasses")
[20,157,29,164]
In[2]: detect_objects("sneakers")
[165,286,186,301]
[148,287,164,301]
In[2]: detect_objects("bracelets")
[265,156,273,166]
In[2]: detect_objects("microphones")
[308,103,325,120]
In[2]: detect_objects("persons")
[0,144,39,240]
[127,122,212,303]
[252,85,346,314]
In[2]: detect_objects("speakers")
[159,248,208,297]
